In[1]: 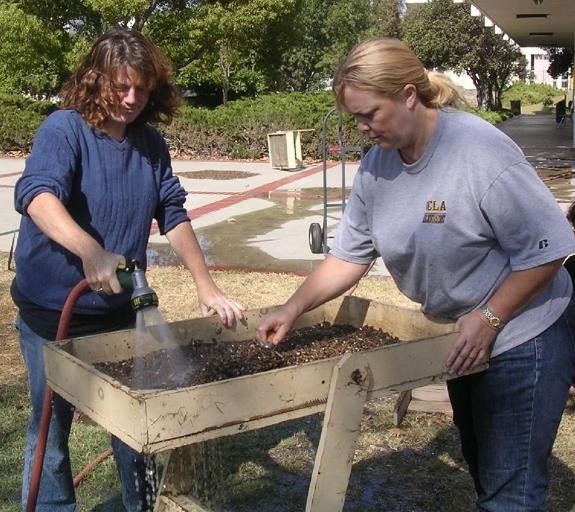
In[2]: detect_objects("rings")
[96,288,102,292]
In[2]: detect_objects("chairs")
[557,102,574,128]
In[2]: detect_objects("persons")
[255,37,575,512]
[10,29,248,512]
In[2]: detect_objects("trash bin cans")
[511,100,521,115]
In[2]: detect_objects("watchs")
[480,305,505,329]
